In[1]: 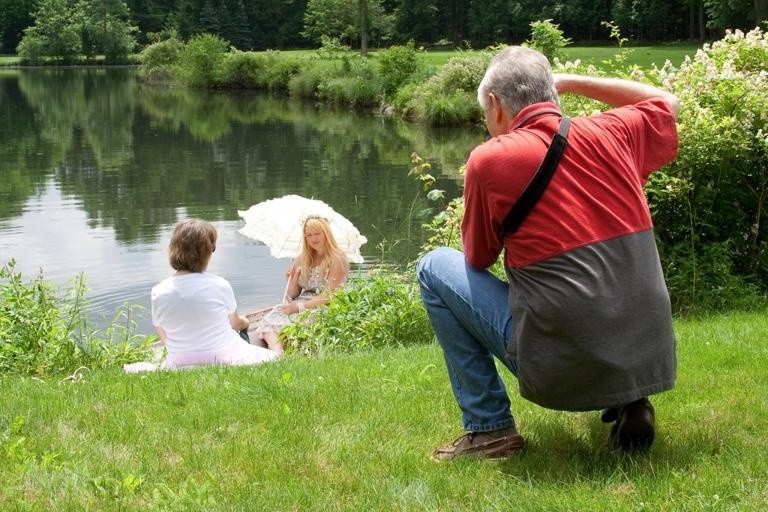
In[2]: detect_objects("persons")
[245,215,349,355]
[417,47,680,464]
[150,219,277,372]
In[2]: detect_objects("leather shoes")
[602,398,656,453]
[430,427,524,461]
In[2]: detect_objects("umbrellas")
[236,195,367,267]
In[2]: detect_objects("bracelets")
[297,302,306,314]
